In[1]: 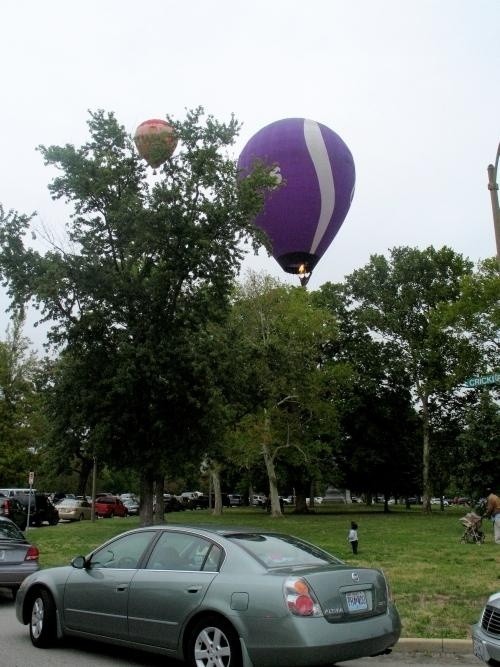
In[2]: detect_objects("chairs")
[152,544,225,572]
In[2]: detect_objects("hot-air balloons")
[135,118,179,176]
[234,115,360,287]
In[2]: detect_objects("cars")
[13,524,402,665]
[94,496,128,519]
[54,498,91,521]
[1,514,41,599]
[153,489,487,514]
[63,491,141,515]
[0,488,61,531]
[471,590,500,663]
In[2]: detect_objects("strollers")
[458,512,489,543]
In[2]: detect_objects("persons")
[266,495,272,512]
[262,493,268,509]
[279,496,285,512]
[480,487,500,544]
[347,520,360,553]
